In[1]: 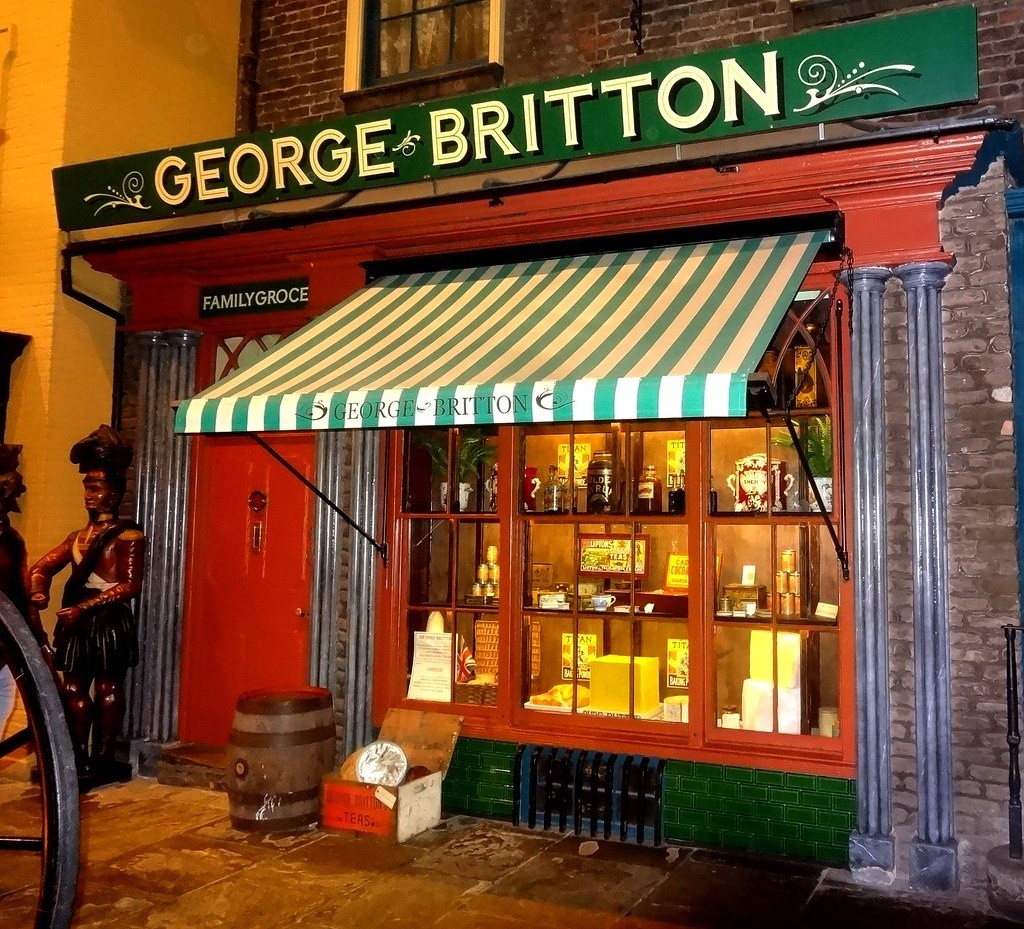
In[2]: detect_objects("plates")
[356,738,407,789]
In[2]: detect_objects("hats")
[70,424,132,474]
[0,443,23,476]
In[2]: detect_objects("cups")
[591,593,616,612]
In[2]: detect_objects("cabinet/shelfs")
[396,303,843,740]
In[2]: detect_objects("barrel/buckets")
[224,685,337,833]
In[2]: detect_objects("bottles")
[541,464,577,515]
[668,470,684,513]
[710,476,716,514]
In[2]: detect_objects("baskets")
[453,619,540,706]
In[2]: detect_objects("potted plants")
[772,413,832,513]
[417,425,498,511]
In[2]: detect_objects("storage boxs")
[316,707,464,841]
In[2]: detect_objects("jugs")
[524,464,539,512]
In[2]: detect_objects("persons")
[29,423,146,773]
[0,443,56,670]
